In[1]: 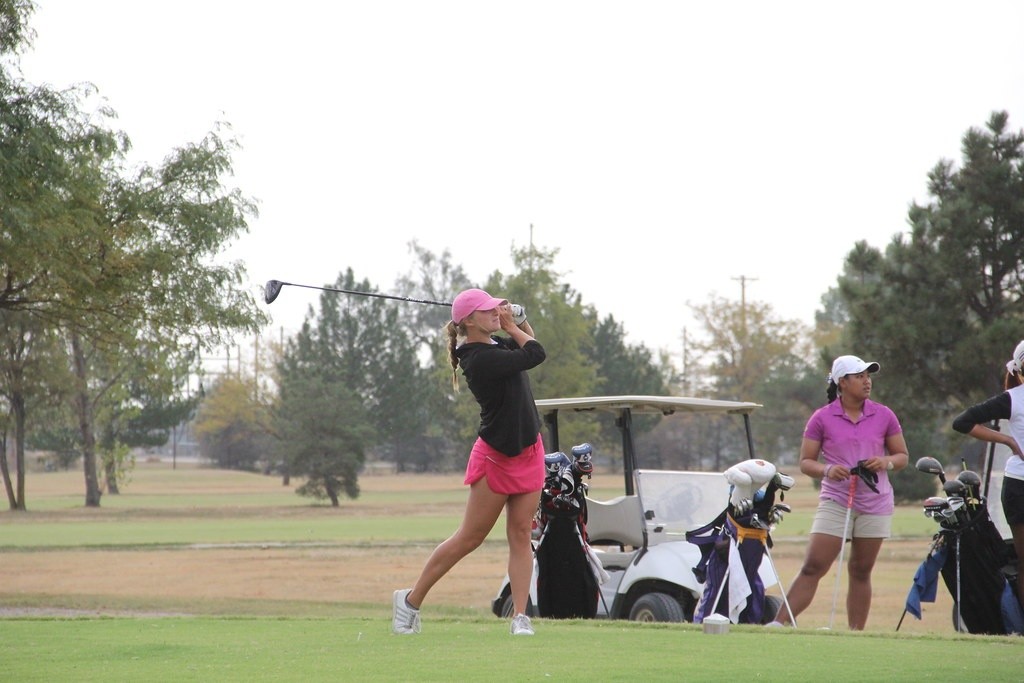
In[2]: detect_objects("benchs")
[582,495,644,569]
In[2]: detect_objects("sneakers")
[391,589,422,635]
[509,613,536,636]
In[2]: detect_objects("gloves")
[510,304,527,326]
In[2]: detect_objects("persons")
[767,355,909,630]
[953,339,1024,608]
[391,289,547,635]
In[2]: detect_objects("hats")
[831,354,881,386]
[1005,339,1024,376]
[451,288,508,327]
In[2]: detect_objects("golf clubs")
[915,455,982,527]
[264,279,524,317]
[817,474,858,631]
[544,442,593,488]
[723,457,795,529]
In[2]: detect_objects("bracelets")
[824,464,832,477]
[887,463,894,471]
